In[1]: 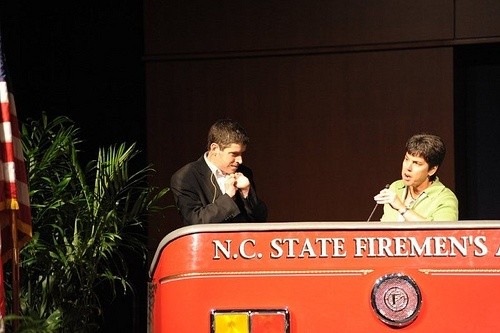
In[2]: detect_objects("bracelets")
[400,207,409,215]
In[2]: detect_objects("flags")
[0,51,32,265]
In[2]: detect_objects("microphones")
[367,184,390,222]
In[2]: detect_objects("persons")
[372,134,459,222]
[170,118,267,225]
